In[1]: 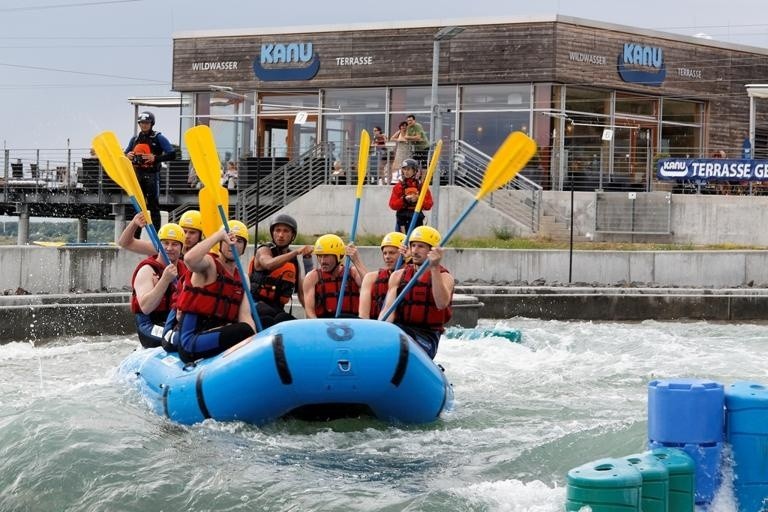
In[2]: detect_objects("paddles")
[334,131,376,320]
[391,138,444,273]
[114,156,179,292]
[34,239,120,249]
[183,122,269,332]
[90,132,174,278]
[193,186,230,259]
[380,130,537,325]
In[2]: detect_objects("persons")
[247,214,315,334]
[388,158,435,235]
[117,209,205,260]
[389,120,415,172]
[370,126,397,185]
[177,219,256,364]
[301,233,370,319]
[123,111,177,240]
[378,225,455,360]
[220,161,238,188]
[330,160,347,185]
[160,271,187,354]
[404,114,432,170]
[358,231,411,321]
[130,222,188,350]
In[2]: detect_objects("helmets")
[269,214,297,250]
[313,233,346,263]
[401,158,419,178]
[409,224,442,248]
[158,223,184,251]
[179,210,202,236]
[381,231,407,251]
[222,219,247,256]
[137,112,155,126]
